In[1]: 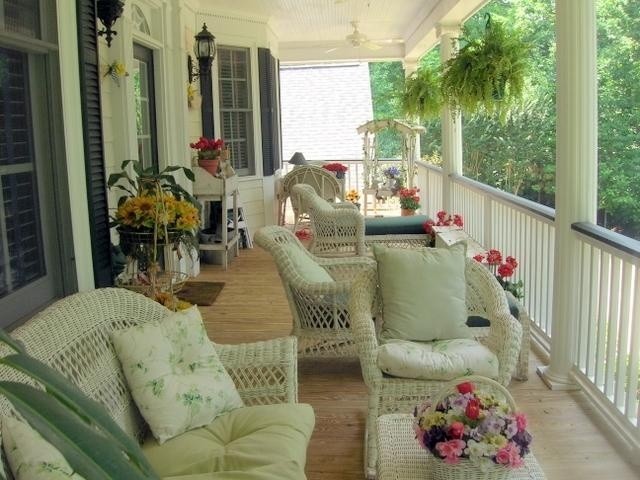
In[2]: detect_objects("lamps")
[188,22,217,82]
[287,153,307,171]
[94,0,125,48]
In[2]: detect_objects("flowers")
[324,163,346,173]
[385,165,406,184]
[398,185,423,209]
[344,187,360,203]
[100,52,128,87]
[410,372,532,471]
[186,86,200,107]
[116,189,202,266]
[424,208,463,242]
[473,248,523,298]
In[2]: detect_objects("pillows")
[373,244,469,341]
[110,306,246,445]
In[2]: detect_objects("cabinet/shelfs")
[432,225,494,270]
[191,156,240,269]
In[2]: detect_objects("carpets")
[177,281,225,304]
[191,138,226,160]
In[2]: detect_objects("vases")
[401,208,416,218]
[199,160,220,177]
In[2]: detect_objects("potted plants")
[391,65,445,122]
[103,159,195,277]
[433,15,538,126]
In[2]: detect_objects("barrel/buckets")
[199,225,234,266]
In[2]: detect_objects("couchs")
[1,284,314,480]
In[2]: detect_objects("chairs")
[258,225,534,379]
[275,167,342,225]
[359,254,522,413]
[294,184,436,248]
[357,118,426,215]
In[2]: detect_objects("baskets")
[417,376,520,480]
